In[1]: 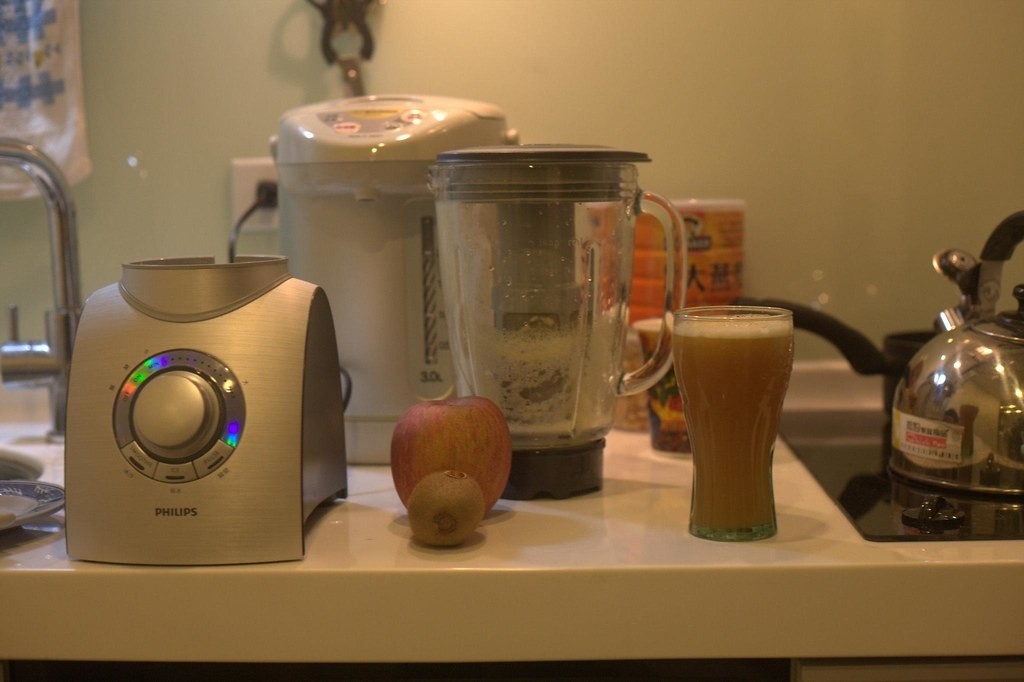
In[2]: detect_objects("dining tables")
[1,380,1023,661]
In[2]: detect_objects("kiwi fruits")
[407,472,485,547]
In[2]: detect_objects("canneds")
[581,195,743,434]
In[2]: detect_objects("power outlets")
[229,157,277,234]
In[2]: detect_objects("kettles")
[889,211,1023,496]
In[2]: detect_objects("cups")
[670,303,793,539]
[633,317,690,455]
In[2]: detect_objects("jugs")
[424,142,687,501]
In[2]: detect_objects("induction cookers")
[775,406,1023,539]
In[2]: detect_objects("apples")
[389,395,512,520]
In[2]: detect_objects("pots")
[757,293,937,423]
